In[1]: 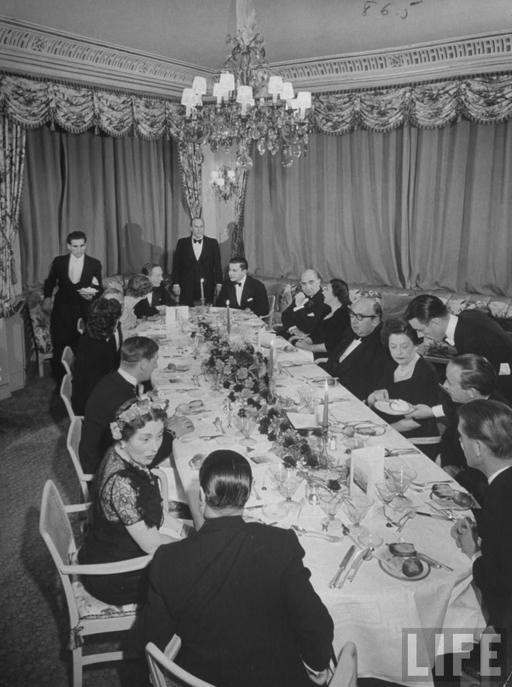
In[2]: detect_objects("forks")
[409,479,454,486]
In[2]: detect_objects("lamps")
[211,164,235,200]
[180,0,312,169]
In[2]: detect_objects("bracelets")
[365,395,368,404]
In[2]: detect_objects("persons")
[43,230,103,365]
[439,351,512,475]
[79,396,196,604]
[434,399,511,686]
[404,293,511,403]
[172,218,225,307]
[81,256,387,489]
[119,448,334,686]
[361,316,441,467]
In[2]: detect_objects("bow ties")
[233,282,241,287]
[355,335,367,343]
[193,240,201,243]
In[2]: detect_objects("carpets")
[0,420,125,686]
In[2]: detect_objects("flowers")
[195,320,316,468]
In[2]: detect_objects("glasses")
[439,383,462,395]
[349,308,375,321]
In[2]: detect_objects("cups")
[135,300,422,558]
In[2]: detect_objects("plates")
[431,489,476,510]
[77,289,100,294]
[379,553,431,580]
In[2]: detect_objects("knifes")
[328,544,355,588]
[415,511,448,520]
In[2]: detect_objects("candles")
[226,300,231,334]
[201,279,204,298]
[270,340,273,376]
[323,379,329,426]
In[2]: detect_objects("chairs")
[407,434,441,468]
[38,316,162,687]
[147,635,358,686]
[260,296,275,325]
